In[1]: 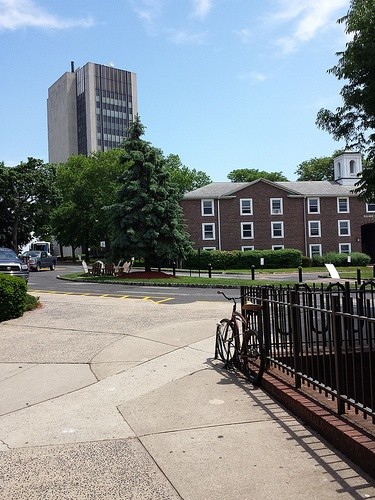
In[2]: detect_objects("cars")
[0,247,29,285]
[19,250,57,272]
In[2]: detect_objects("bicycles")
[216,290,266,384]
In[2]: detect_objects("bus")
[28,241,51,255]
